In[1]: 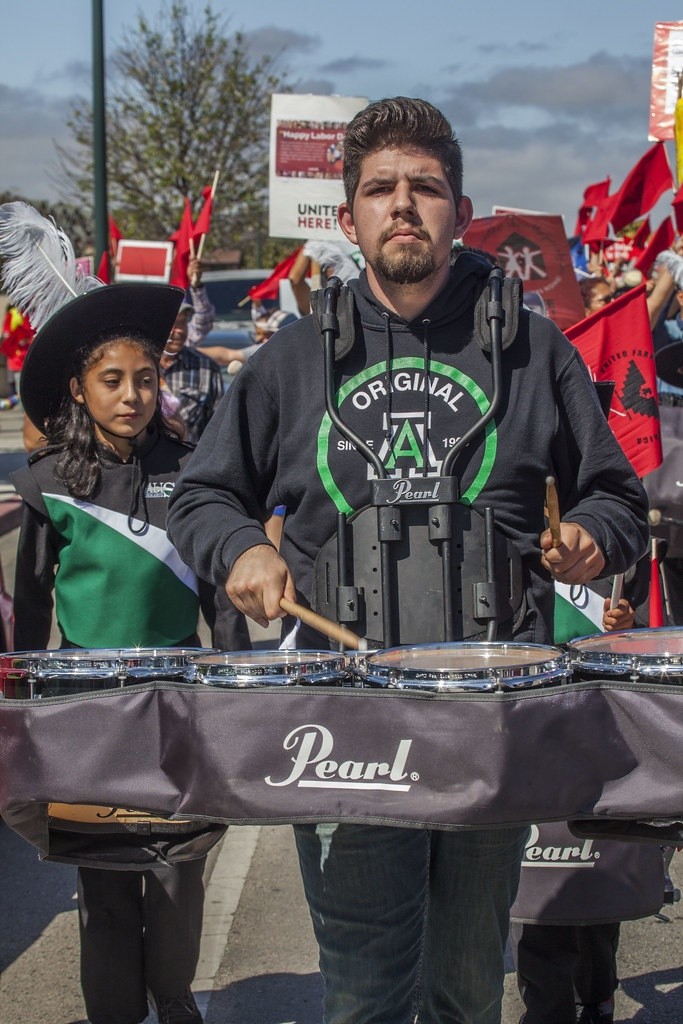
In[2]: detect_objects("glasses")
[594,290,616,302]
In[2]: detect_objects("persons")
[160,243,312,456]
[165,95,648,1024]
[4,301,35,398]
[12,281,255,1024]
[551,247,683,644]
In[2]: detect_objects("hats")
[0,200,187,434]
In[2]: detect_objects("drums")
[0,644,222,702]
[353,639,576,695]
[184,648,353,691]
[570,623,683,829]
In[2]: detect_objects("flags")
[560,283,663,479]
[575,143,683,273]
[168,184,213,287]
[250,246,302,300]
[97,216,121,285]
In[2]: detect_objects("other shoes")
[145,981,206,1024]
